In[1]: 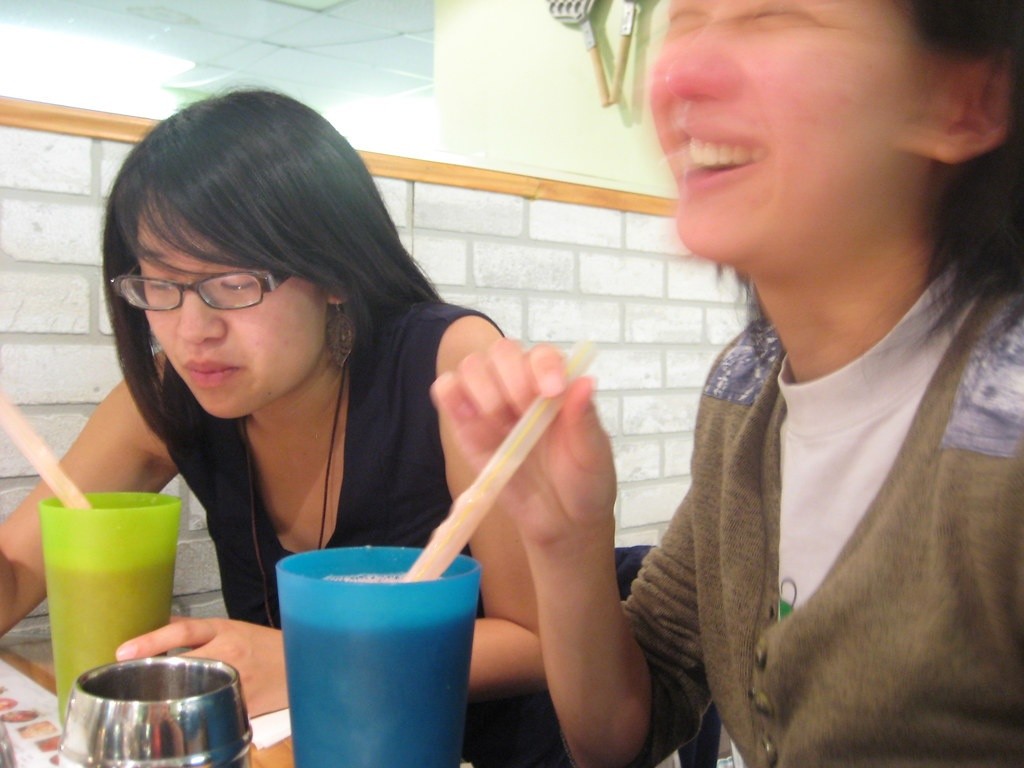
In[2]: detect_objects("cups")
[37,492,181,732]
[276,546,481,768]
[62,657,252,768]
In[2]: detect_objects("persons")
[1,89,573,768]
[427,0,1024,768]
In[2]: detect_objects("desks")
[1,638,475,767]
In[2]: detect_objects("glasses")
[109,261,292,310]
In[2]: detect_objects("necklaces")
[244,356,347,629]
